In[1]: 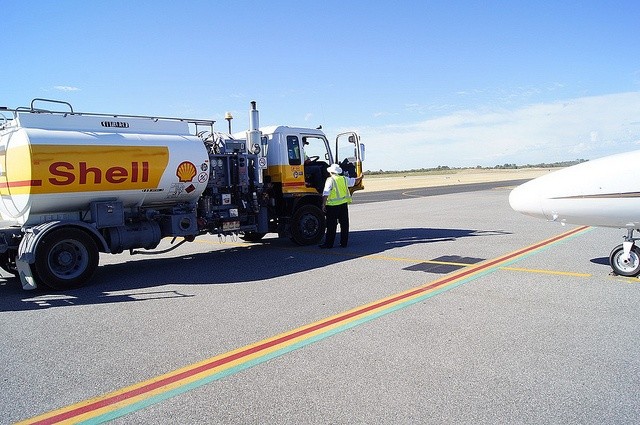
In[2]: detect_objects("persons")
[318,164,364,249]
[293,137,324,193]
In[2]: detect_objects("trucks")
[0,97,365,292]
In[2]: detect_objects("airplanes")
[508,148,640,277]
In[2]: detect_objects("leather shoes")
[338,244,345,248]
[318,244,331,249]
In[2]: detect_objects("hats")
[326,164,342,174]
[303,137,309,145]
